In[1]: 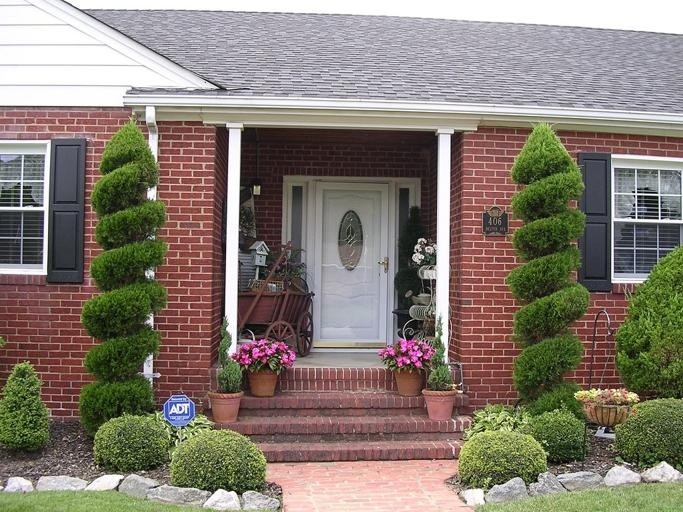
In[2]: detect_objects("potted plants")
[391,206,435,341]
[207,316,245,423]
[573,387,639,406]
[238,206,256,292]
[421,364,456,420]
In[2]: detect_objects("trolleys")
[237,240,315,357]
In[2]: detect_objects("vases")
[246,369,278,397]
[393,368,423,396]
[583,405,627,426]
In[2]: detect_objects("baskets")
[584,403,630,427]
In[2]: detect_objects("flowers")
[229,339,295,376]
[411,237,436,265]
[377,338,434,375]
